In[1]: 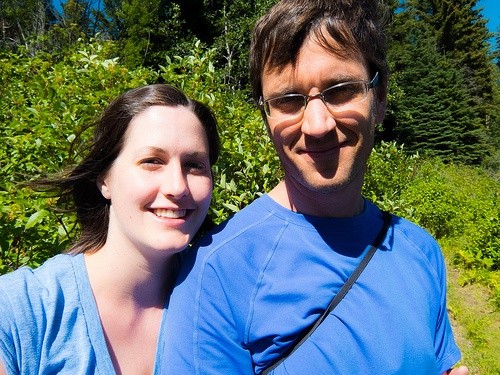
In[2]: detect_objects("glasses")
[258,72,381,119]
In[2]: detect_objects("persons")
[154,0,467,375]
[0,84,222,375]
